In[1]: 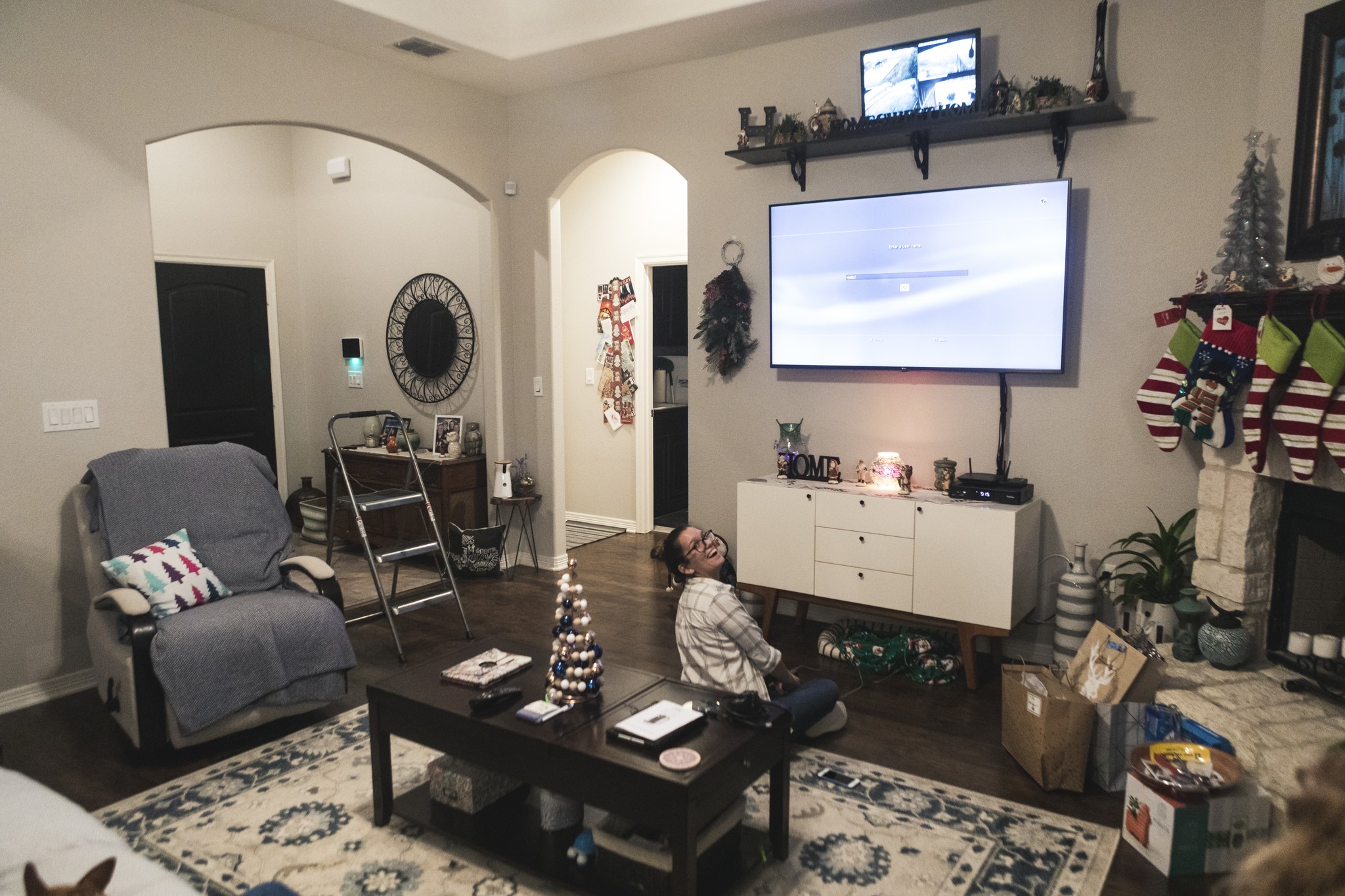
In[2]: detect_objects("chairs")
[71,441,357,755]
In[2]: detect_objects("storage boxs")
[592,796,748,896]
[428,754,523,816]
[1121,771,1273,878]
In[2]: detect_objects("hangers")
[566,520,627,551]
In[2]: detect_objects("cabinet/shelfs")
[655,407,688,521]
[736,475,1041,689]
[322,445,486,566]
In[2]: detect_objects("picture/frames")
[433,415,463,455]
[379,416,412,446]
[1282,0,1345,261]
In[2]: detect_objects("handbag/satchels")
[1000,615,1181,795]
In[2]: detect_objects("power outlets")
[1066,562,1073,573]
[1098,562,1116,591]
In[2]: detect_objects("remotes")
[470,686,522,709]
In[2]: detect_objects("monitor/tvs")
[768,174,1073,374]
[858,27,984,121]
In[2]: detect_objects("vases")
[511,476,535,497]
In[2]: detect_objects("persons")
[651,524,840,740]
[1215,740,1345,896]
[856,459,867,487]
[898,464,912,493]
[828,460,839,484]
[437,420,460,453]
[778,455,788,479]
[943,468,951,496]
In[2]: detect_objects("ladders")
[326,410,475,661]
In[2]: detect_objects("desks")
[490,494,542,580]
[367,640,793,896]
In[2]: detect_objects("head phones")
[717,691,797,731]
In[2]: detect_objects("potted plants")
[1094,503,1202,642]
[1020,75,1080,118]
[774,113,808,147]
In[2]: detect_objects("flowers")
[510,454,529,475]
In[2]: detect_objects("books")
[441,647,533,689]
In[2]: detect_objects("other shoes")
[802,701,846,738]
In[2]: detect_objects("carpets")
[91,701,1117,896]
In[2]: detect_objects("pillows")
[442,522,507,578]
[100,527,234,619]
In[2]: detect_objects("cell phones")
[816,768,860,789]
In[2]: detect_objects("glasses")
[681,530,716,563]
